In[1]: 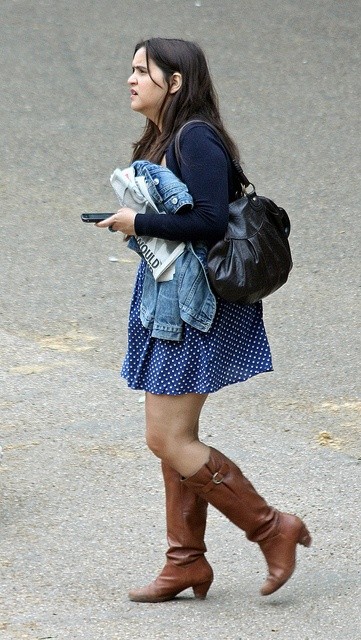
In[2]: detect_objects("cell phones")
[80,212,116,223]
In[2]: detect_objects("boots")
[128,459,214,603]
[181,446,313,597]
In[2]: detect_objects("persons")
[95,37,312,603]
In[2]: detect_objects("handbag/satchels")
[174,121,291,306]
